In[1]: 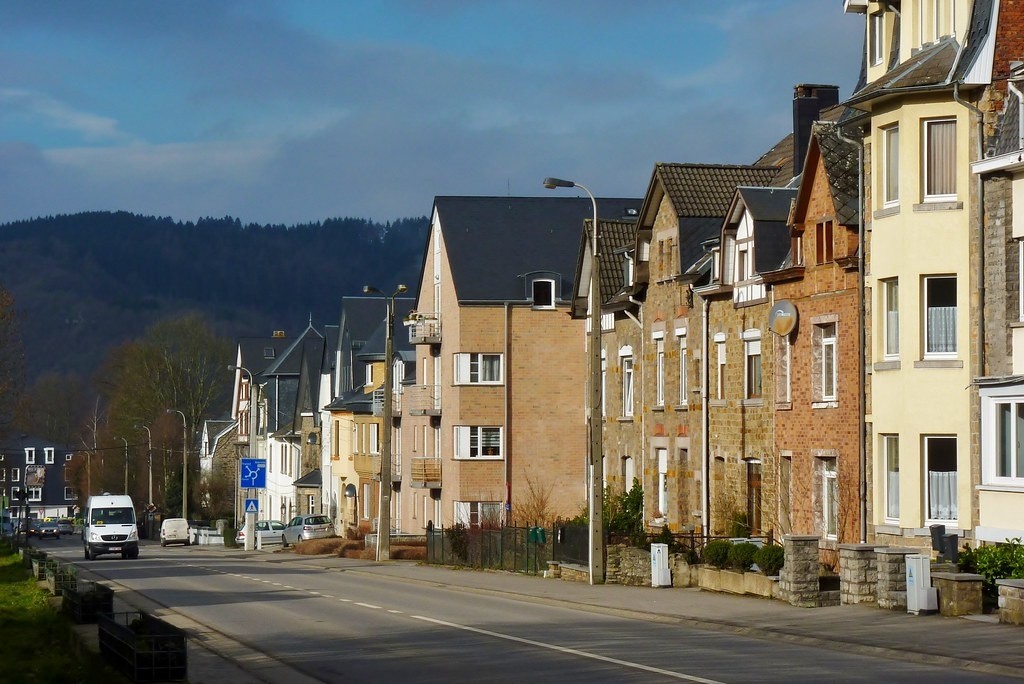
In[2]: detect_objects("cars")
[38,522,60,540]
[56,520,74,535]
[27,526,40,536]
[236,520,286,546]
[281,515,335,547]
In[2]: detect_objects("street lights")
[167,409,188,518]
[543,176,606,585]
[135,424,153,539]
[362,284,408,561]
[114,436,127,496]
[227,365,256,551]
[78,449,91,498]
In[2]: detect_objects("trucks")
[83,492,140,559]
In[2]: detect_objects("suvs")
[158,518,190,546]
